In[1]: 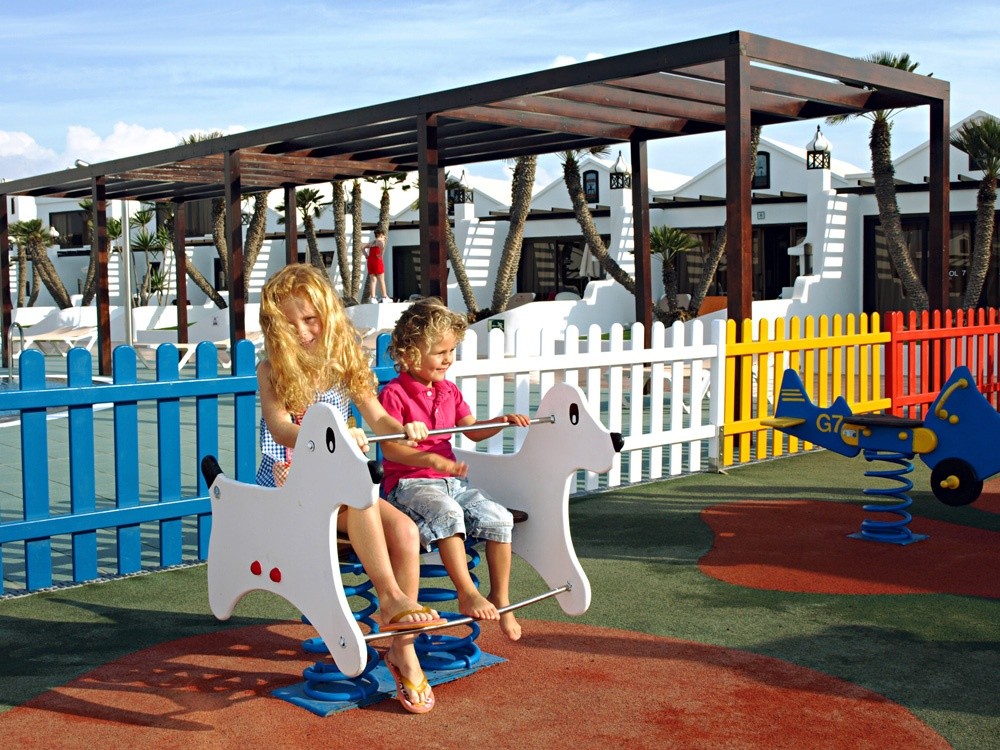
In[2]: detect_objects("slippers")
[384,652,435,713]
[379,605,446,632]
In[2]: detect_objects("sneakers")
[370,298,378,304]
[382,296,393,303]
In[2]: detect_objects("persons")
[258,264,447,715]
[378,298,521,642]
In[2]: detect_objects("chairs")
[6,287,416,376]
